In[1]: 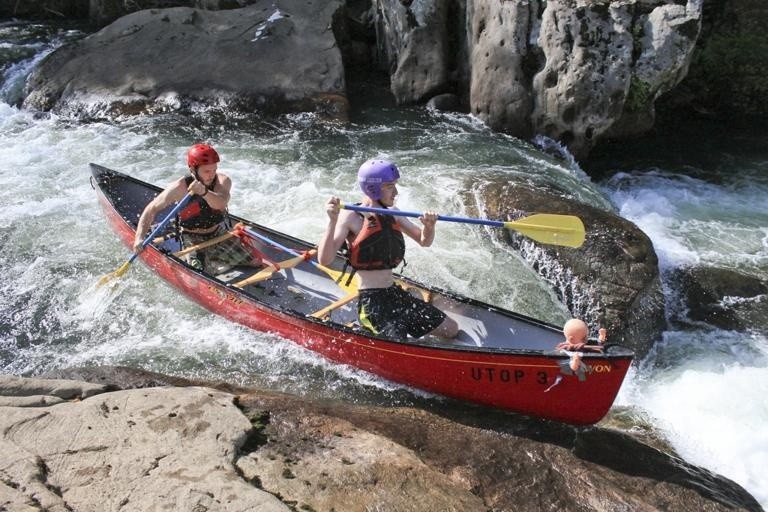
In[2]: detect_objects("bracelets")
[199,187,208,197]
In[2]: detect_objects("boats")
[88,162,634,429]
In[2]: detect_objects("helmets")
[358,157,398,201]
[187,144,220,176]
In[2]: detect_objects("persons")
[316,158,460,347]
[131,143,263,278]
[554,318,607,352]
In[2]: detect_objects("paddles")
[92,189,194,291]
[235,224,362,295]
[337,204,586,249]
